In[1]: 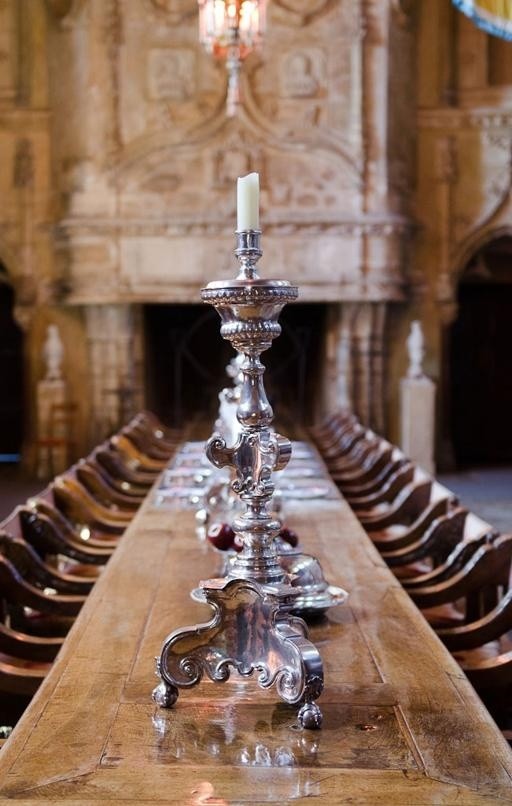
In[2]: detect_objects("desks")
[0,442,511,806]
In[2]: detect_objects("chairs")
[359,480,460,552]
[0,556,89,661]
[57,414,184,520]
[0,657,50,746]
[405,536,512,661]
[26,485,131,548]
[381,509,502,585]
[312,412,416,506]
[0,506,118,596]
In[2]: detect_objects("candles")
[237,175,258,231]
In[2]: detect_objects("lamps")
[197,0,265,107]
